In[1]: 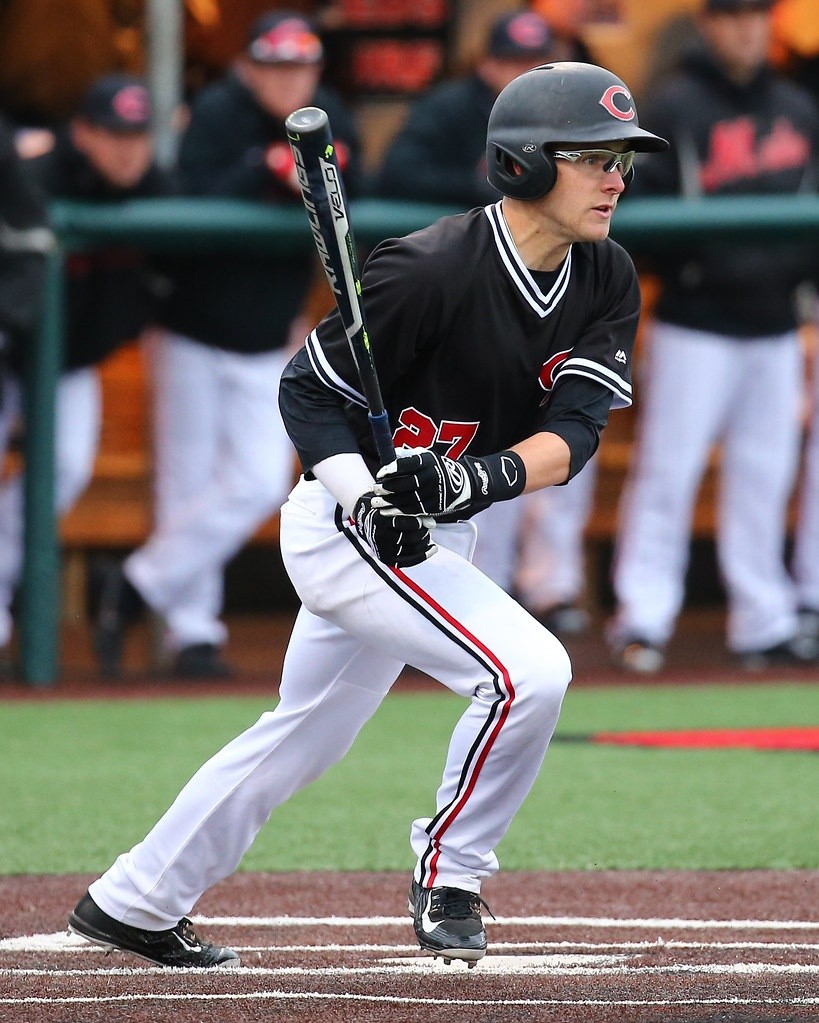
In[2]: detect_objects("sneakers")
[407,869,495,969]
[66,890,241,969]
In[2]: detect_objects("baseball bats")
[283,106,397,478]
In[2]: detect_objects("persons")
[1,71,197,684]
[82,13,357,683]
[362,12,618,640]
[66,61,671,971]
[602,0,819,685]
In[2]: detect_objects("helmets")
[486,61,671,200]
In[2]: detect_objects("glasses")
[552,148,635,179]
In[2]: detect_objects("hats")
[80,73,152,134]
[486,6,558,60]
[246,7,325,64]
[704,1,777,14]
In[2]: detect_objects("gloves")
[370,446,527,524]
[350,489,438,569]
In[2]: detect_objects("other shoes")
[162,644,239,693]
[82,546,149,685]
[734,635,819,667]
[615,637,666,678]
